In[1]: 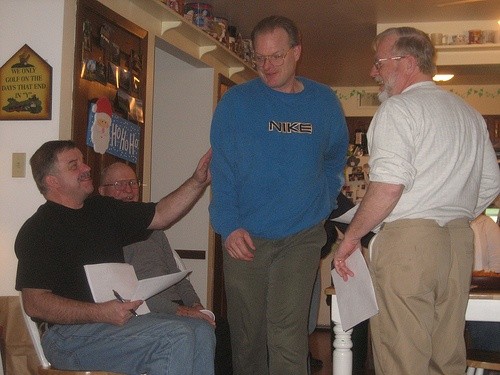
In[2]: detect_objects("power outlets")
[11,153,26,178]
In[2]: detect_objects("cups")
[431,33,443,46]
[469,30,481,44]
[482,31,493,44]
[201,309,215,321]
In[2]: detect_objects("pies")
[473,269,500,276]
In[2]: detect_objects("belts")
[40,322,53,334]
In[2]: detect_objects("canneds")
[162,0,257,70]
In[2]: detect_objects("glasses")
[100,178,141,191]
[252,44,294,66]
[372,55,406,72]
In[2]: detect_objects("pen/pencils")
[113,290,139,317]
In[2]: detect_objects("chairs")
[18,291,127,375]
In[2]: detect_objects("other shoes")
[310,357,323,366]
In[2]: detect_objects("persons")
[98,161,232,375]
[334,27,500,375]
[15,140,216,375]
[210,17,348,375]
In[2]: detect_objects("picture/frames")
[71,0,148,202]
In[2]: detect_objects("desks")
[324,285,500,375]
